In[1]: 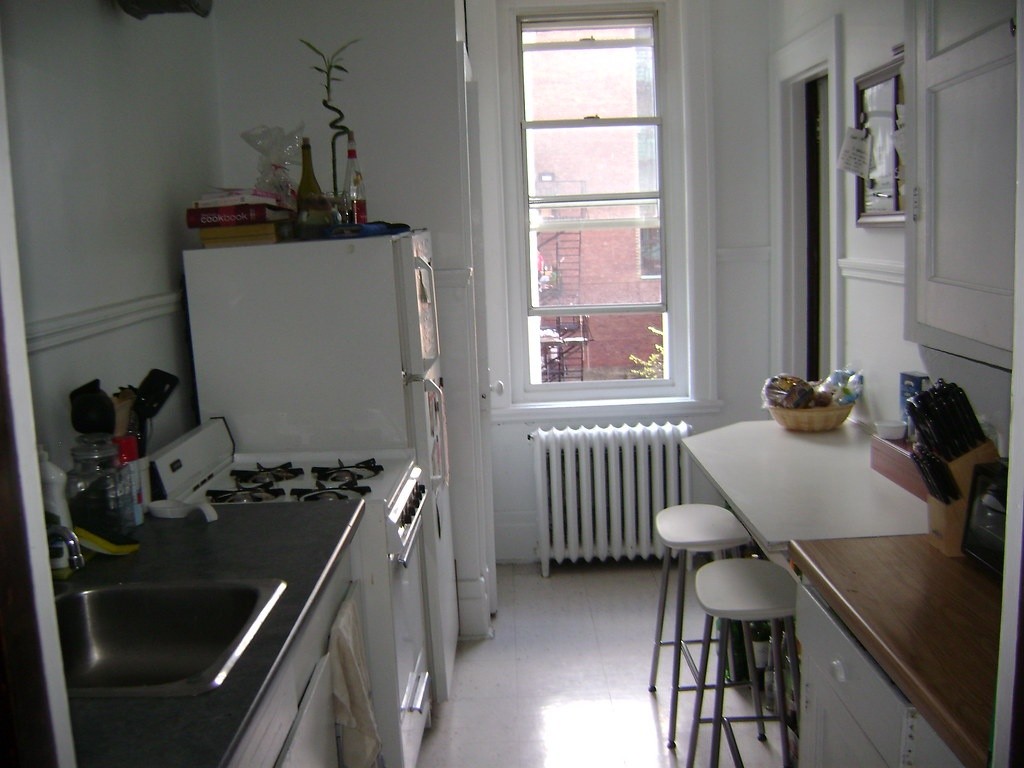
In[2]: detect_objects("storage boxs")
[871,432,927,502]
[527,420,694,578]
[200,221,285,248]
[899,371,930,435]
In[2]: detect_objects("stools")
[648,504,768,749]
[686,557,797,768]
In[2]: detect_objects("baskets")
[761,378,856,432]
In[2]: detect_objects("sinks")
[52,577,287,696]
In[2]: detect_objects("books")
[185,188,296,229]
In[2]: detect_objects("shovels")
[132,369,179,457]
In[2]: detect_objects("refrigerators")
[182,228,459,711]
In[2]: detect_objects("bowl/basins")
[875,420,907,440]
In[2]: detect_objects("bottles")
[341,130,368,225]
[296,138,324,233]
[716,553,801,741]
[66,431,151,534]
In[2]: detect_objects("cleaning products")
[36,442,75,532]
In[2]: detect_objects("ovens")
[361,512,435,768]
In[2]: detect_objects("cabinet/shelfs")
[796,574,965,768]
[903,0,1023,370]
[223,541,387,768]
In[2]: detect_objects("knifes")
[907,378,988,505]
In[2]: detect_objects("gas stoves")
[147,417,429,554]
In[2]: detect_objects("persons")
[537,249,553,292]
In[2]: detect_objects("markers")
[873,192,891,198]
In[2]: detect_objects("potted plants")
[297,35,364,227]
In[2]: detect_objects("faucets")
[45,523,87,573]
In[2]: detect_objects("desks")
[682,421,928,682]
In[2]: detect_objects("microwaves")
[961,461,1008,585]
